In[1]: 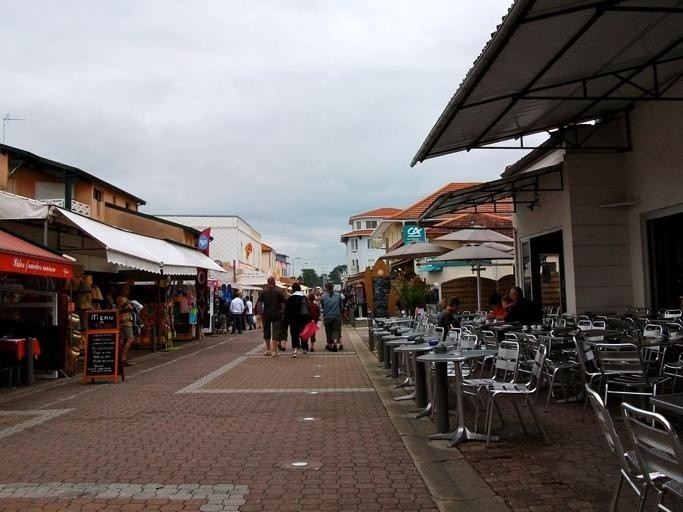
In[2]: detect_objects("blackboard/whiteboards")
[85,309,119,330]
[83,330,119,377]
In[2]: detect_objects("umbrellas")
[432,224,515,244]
[378,241,455,263]
[434,243,514,313]
[479,242,514,253]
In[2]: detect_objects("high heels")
[325,345,332,351]
[339,344,344,352]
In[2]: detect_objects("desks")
[0,335,42,386]
[367,318,499,449]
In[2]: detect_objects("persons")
[116,284,134,368]
[485,286,543,334]
[218,277,344,357]
[436,296,460,341]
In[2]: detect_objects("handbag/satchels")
[298,322,320,341]
[300,299,309,315]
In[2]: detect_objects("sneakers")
[271,352,281,357]
[122,360,134,367]
[263,351,271,356]
